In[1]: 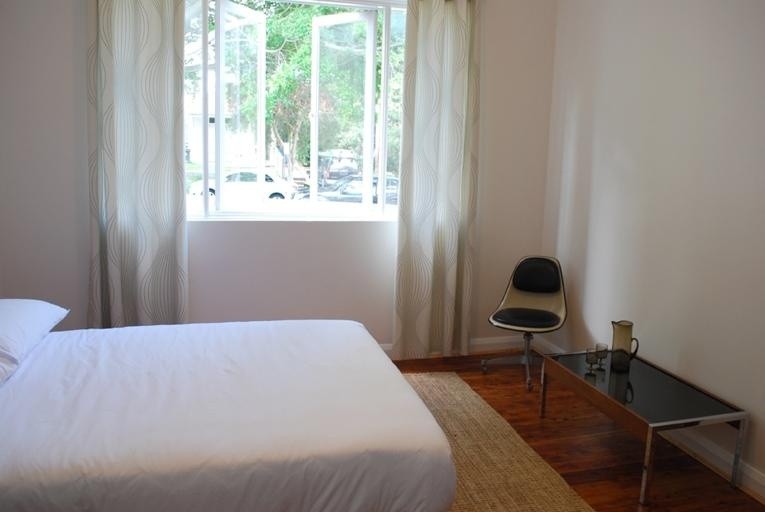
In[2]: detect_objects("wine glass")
[595,344,609,371]
[587,348,598,370]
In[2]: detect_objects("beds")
[0,319,456,512]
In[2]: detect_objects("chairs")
[480,255,568,392]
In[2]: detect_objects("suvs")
[317,153,359,186]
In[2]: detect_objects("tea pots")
[611,320,639,374]
[610,373,634,406]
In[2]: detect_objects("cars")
[295,173,400,204]
[190,167,295,201]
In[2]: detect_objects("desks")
[539,348,751,506]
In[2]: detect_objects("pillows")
[0,298,71,386]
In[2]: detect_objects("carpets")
[401,371,596,512]
